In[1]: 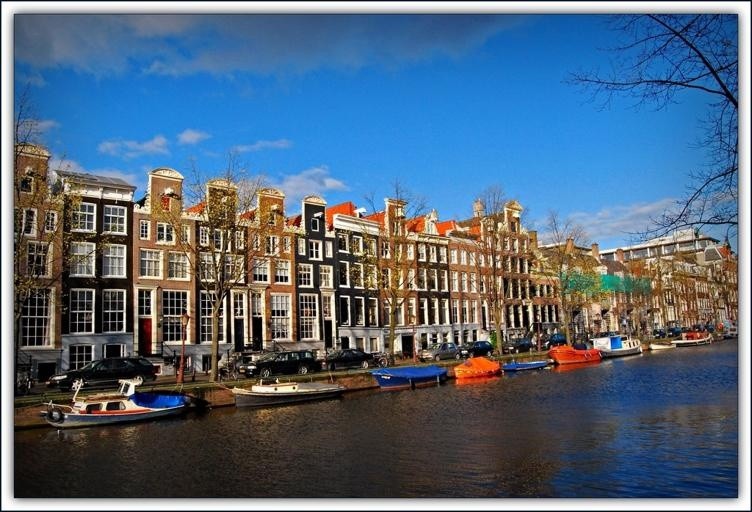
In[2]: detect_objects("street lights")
[535,313,543,353]
[175,311,192,387]
[643,313,648,336]
[709,311,713,322]
[595,313,602,335]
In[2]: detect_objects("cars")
[650,323,715,340]
[537,332,566,351]
[592,330,616,337]
[417,341,461,362]
[458,340,494,358]
[501,337,532,354]
[240,349,317,378]
[316,349,375,371]
[45,356,159,390]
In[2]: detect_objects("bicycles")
[16,367,32,393]
[369,351,394,368]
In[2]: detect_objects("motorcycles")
[219,361,241,381]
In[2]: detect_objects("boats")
[500,359,550,371]
[450,355,501,378]
[504,370,525,379]
[545,343,602,362]
[37,375,198,427]
[365,363,449,391]
[232,376,345,409]
[587,335,642,359]
[455,376,500,388]
[649,332,714,349]
[550,361,599,373]
